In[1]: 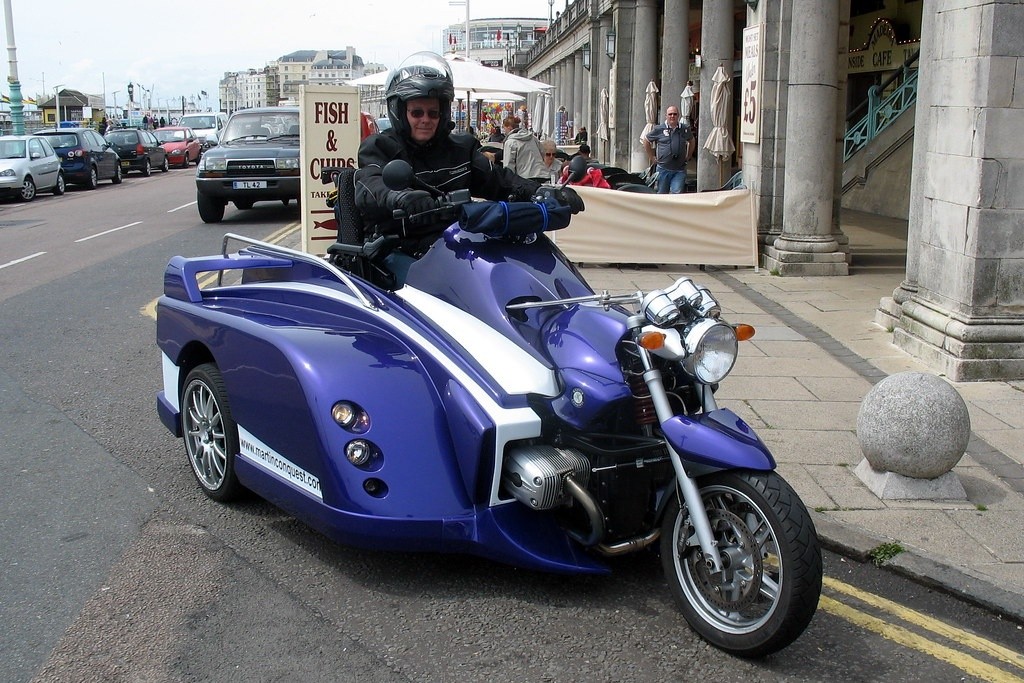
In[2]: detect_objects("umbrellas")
[596,87,609,165]
[343,53,557,142]
[639,62,736,189]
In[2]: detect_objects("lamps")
[605,31,616,61]
[582,43,591,71]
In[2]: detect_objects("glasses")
[668,112,677,116]
[546,153,556,156]
[407,107,443,120]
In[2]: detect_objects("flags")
[0,94,36,105]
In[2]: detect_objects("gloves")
[394,190,438,224]
[536,184,585,215]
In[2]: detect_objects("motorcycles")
[156,160,822,657]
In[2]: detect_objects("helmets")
[384,65,455,152]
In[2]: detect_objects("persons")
[142,114,178,132]
[353,66,585,292]
[99,115,121,136]
[643,105,695,194]
[456,103,611,189]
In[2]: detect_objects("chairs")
[128,135,138,143]
[327,170,396,287]
[253,127,271,137]
[601,166,660,195]
[289,125,299,135]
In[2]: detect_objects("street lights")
[448,0,470,132]
[112,91,120,120]
[516,24,522,50]
[548,0,555,24]
[505,44,509,62]
[507,41,512,61]
[51,84,64,128]
[514,31,518,53]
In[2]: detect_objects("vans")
[173,112,229,157]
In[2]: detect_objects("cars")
[196,106,300,223]
[23,128,122,189]
[471,120,477,129]
[0,135,66,202]
[152,127,201,168]
[377,117,392,134]
[103,128,169,176]
[117,119,130,128]
